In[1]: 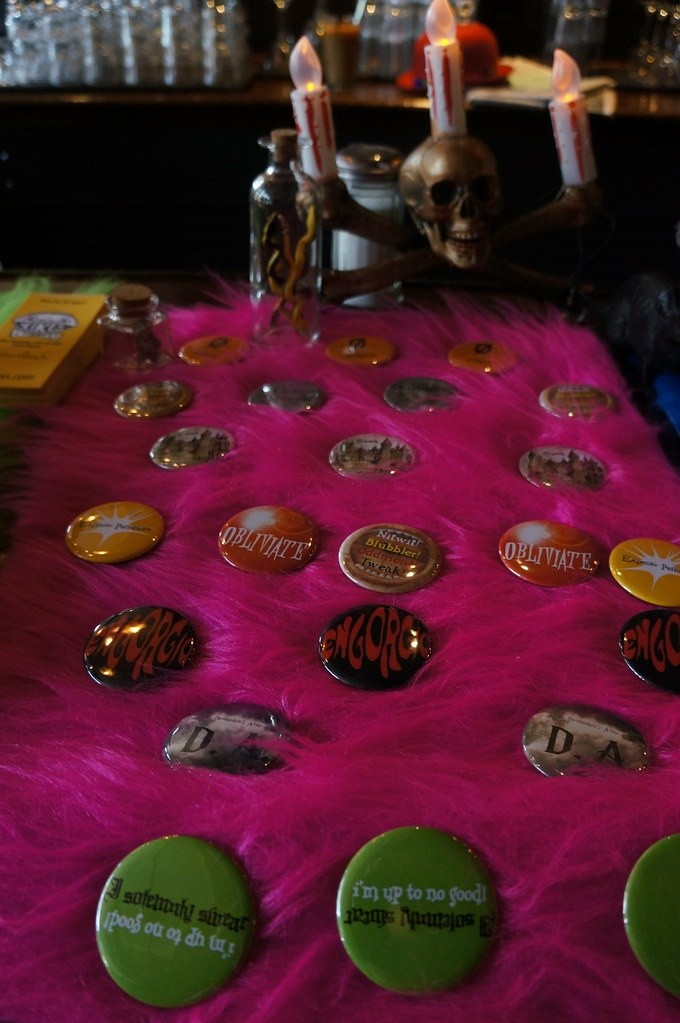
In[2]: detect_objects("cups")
[316,1,428,90]
[0,1,249,86]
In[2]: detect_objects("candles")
[290,36,338,178]
[425,0,469,137]
[548,47,600,187]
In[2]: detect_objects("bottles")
[249,129,324,348]
[262,1,296,80]
[544,1,609,70]
[94,283,171,374]
[332,144,407,308]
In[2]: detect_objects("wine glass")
[625,1,680,88]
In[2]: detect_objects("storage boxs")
[0,291,115,407]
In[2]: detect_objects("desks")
[0,52,680,287]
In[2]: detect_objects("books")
[0,293,112,403]
